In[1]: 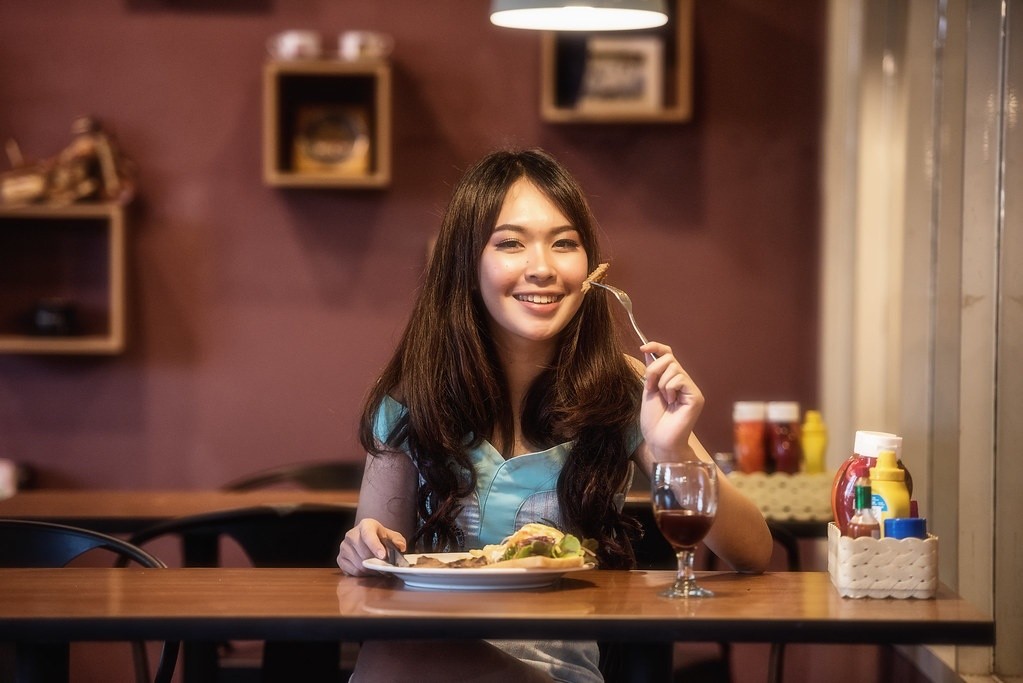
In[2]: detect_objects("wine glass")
[651,460,718,598]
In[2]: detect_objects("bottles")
[831,431,913,537]
[715,452,733,474]
[767,402,802,473]
[885,518,926,540]
[869,451,910,537]
[799,410,828,474]
[846,486,881,540]
[847,466,871,523]
[733,402,766,474]
[64,115,121,205]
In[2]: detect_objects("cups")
[340,31,394,62]
[266,31,322,61]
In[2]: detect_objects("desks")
[1,486,830,569]
[0,567,996,683]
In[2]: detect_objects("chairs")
[0,457,799,682]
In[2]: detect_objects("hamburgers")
[480,523,585,568]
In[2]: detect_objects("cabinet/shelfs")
[541,0,694,123]
[0,201,132,351]
[262,59,392,187]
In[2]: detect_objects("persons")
[334,151,775,683]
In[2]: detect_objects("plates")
[361,553,584,589]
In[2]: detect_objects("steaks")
[412,556,488,568]
[581,263,610,294]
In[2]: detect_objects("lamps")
[487,0,668,31]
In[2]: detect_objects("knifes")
[379,538,410,567]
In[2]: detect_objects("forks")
[589,283,659,361]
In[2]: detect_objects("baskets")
[827,523,940,599]
